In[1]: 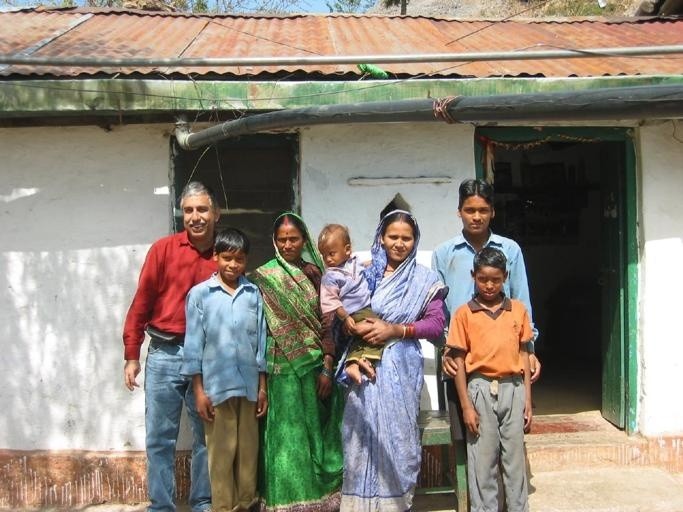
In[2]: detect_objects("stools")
[418,409,469,511]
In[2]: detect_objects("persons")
[318,224,383,385]
[424,177,541,512]
[246,211,346,512]
[121,181,219,512]
[180,226,269,512]
[334,192,450,512]
[446,247,533,512]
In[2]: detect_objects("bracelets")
[320,366,334,379]
[259,387,268,396]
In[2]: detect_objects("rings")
[371,337,374,343]
